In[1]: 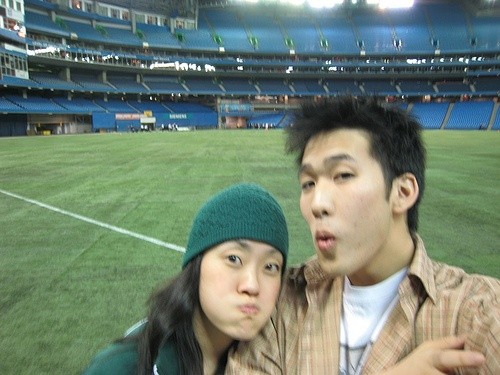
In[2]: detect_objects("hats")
[181,182,289,270]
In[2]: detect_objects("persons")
[84,183,290,375]
[224,96,500,375]
[129,123,275,132]
